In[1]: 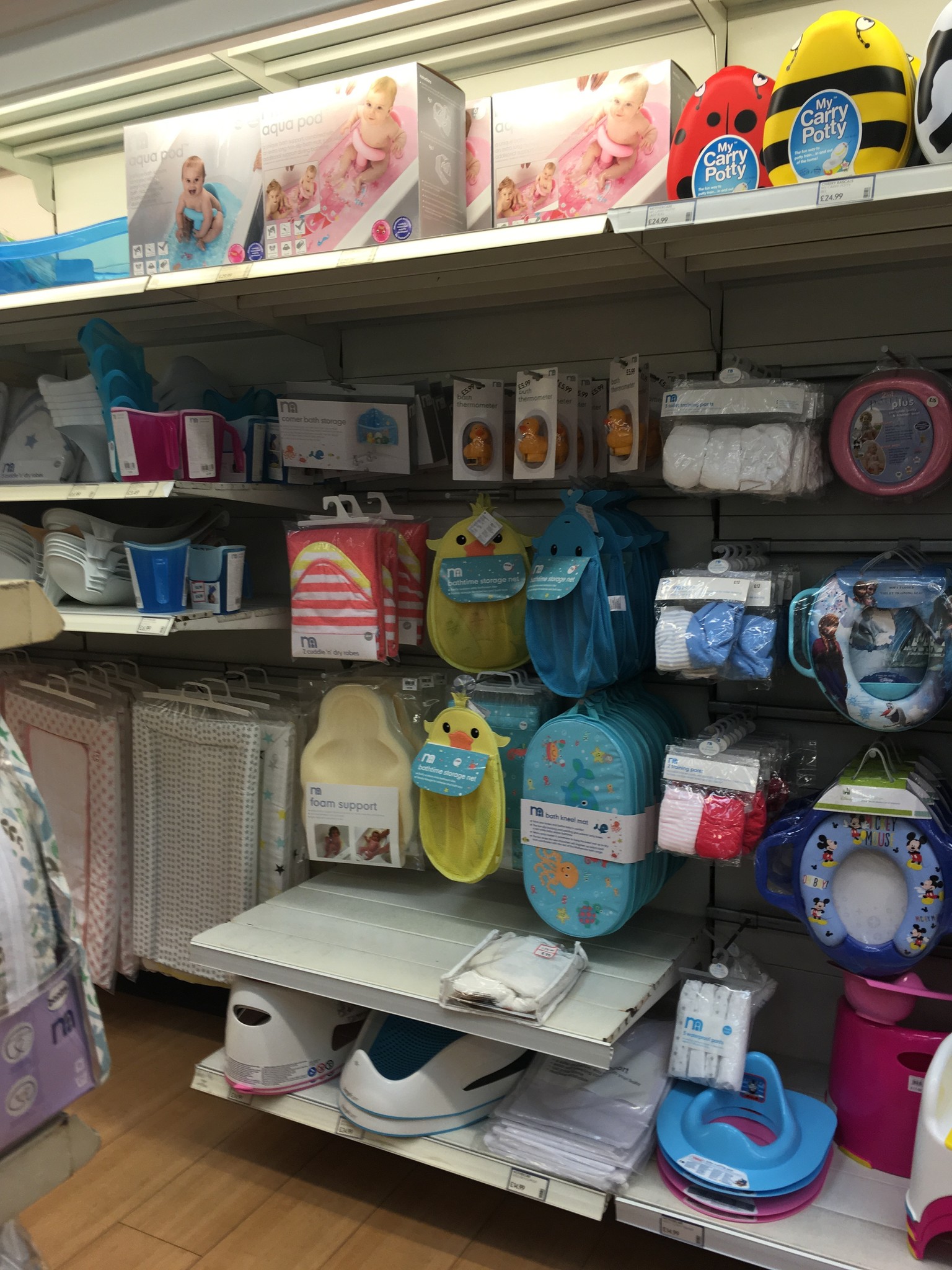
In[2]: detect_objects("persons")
[328,76,408,196]
[527,162,556,209]
[266,179,295,220]
[175,156,223,252]
[466,111,480,181]
[292,165,317,211]
[577,73,658,191]
[577,71,609,92]
[497,176,527,219]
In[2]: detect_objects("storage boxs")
[124,56,692,275]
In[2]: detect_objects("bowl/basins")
[0,507,229,607]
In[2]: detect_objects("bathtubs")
[498,82,671,229]
[467,117,493,232]
[265,87,417,262]
[129,122,262,277]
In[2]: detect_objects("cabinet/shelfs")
[0,0,952,1270]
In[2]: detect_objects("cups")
[191,545,245,614]
[124,537,192,613]
